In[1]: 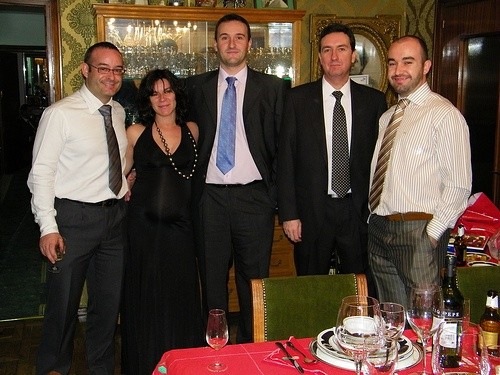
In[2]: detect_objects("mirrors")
[309,13,403,108]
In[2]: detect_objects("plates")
[308,327,423,372]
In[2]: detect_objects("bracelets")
[155,119,199,179]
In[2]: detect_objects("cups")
[479,345,500,375]
[362,302,406,375]
[430,320,492,375]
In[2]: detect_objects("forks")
[286,341,320,365]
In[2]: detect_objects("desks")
[153,325,500,375]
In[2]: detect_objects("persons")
[27,41,128,375]
[121,68,207,375]
[183,13,284,345]
[365,35,473,330]
[277,24,386,274]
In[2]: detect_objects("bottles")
[432,255,465,368]
[454,225,468,267]
[475,290,500,357]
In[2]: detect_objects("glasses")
[86,61,126,75]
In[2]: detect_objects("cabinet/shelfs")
[91,3,307,86]
[228,213,300,312]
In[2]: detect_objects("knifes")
[275,342,305,375]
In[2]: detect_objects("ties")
[368,99,411,213]
[331,91,351,200]
[216,77,238,175]
[97,105,123,197]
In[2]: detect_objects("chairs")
[454,265,500,324]
[250,274,368,343]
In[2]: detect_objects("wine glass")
[205,309,229,372]
[406,284,444,375]
[335,296,385,375]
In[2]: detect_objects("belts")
[388,211,433,221]
[93,198,119,207]
